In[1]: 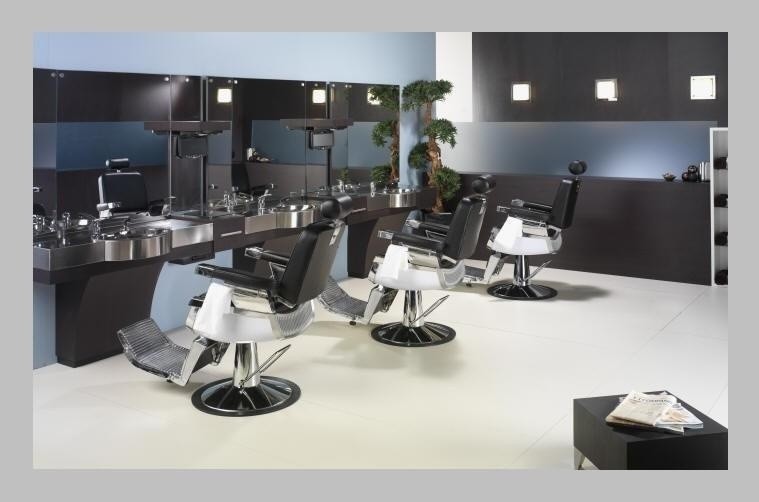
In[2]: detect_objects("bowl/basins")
[662,173,676,182]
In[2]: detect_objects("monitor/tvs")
[177,134,209,158]
[310,131,334,150]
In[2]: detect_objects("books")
[603,391,704,436]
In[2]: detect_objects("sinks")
[105,225,170,240]
[384,188,415,195]
[257,193,273,208]
[269,204,314,212]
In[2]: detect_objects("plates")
[256,158,274,163]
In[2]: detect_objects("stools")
[573,390,728,470]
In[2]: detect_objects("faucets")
[369,180,382,192]
[162,196,176,216]
[336,179,345,192]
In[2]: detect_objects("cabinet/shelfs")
[709,127,728,286]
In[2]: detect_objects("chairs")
[97,158,148,218]
[317,173,497,348]
[460,160,587,301]
[117,193,354,417]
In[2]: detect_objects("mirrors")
[33,119,400,238]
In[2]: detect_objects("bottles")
[339,180,345,192]
[246,144,256,161]
[370,182,377,194]
[223,191,229,206]
[226,202,233,215]
